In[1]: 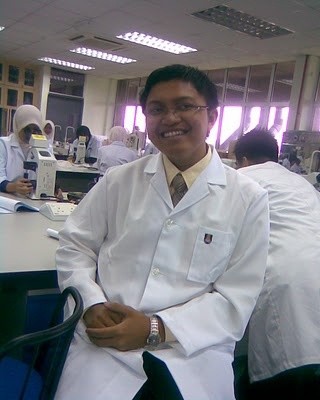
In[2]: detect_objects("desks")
[0,191,68,356]
[57,160,99,178]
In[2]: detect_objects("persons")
[73,126,101,163]
[54,63,269,400]
[0,105,63,200]
[42,120,55,144]
[233,128,320,400]
[97,126,136,180]
[281,153,290,168]
[289,159,302,173]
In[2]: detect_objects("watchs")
[146,316,160,345]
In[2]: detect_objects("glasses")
[145,104,209,119]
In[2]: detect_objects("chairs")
[0,286,83,400]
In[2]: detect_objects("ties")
[171,174,189,207]
